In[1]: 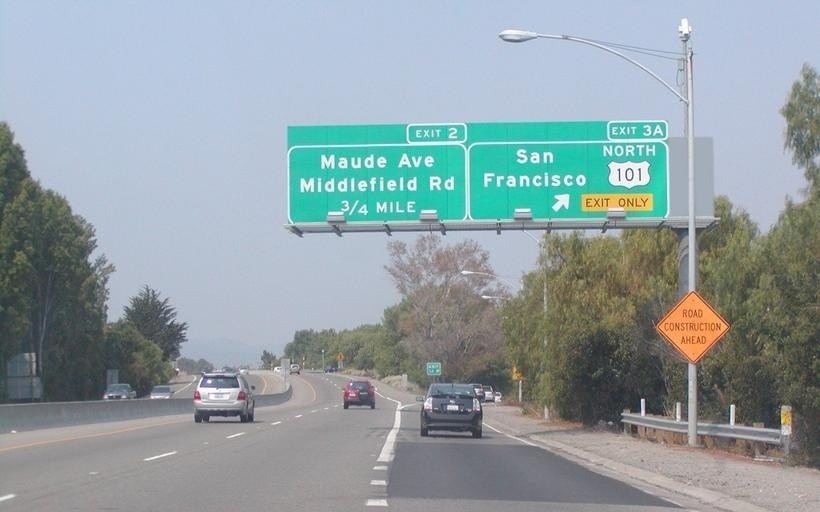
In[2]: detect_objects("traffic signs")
[287,120,668,223]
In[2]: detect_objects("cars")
[274,364,300,376]
[150,385,175,399]
[344,381,374,409]
[193,366,255,421]
[102,384,137,399]
[416,383,502,438]
[323,366,337,374]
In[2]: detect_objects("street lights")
[461,271,523,302]
[499,28,698,445]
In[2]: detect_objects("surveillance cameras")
[680,16,691,39]
